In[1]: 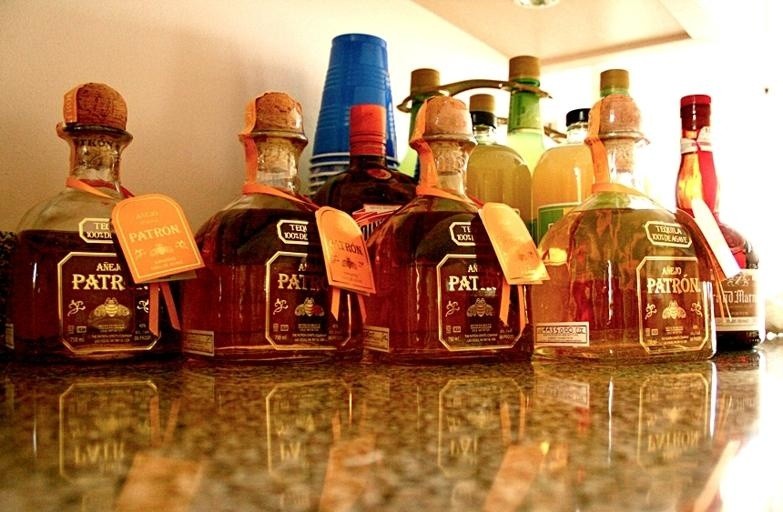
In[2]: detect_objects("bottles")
[499,54,564,235]
[528,109,595,244]
[167,92,355,367]
[313,103,416,351]
[465,93,505,206]
[12,347,765,512]
[397,68,446,183]
[526,69,716,367]
[671,94,763,352]
[7,82,164,372]
[355,95,529,364]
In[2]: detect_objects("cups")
[305,32,401,196]
